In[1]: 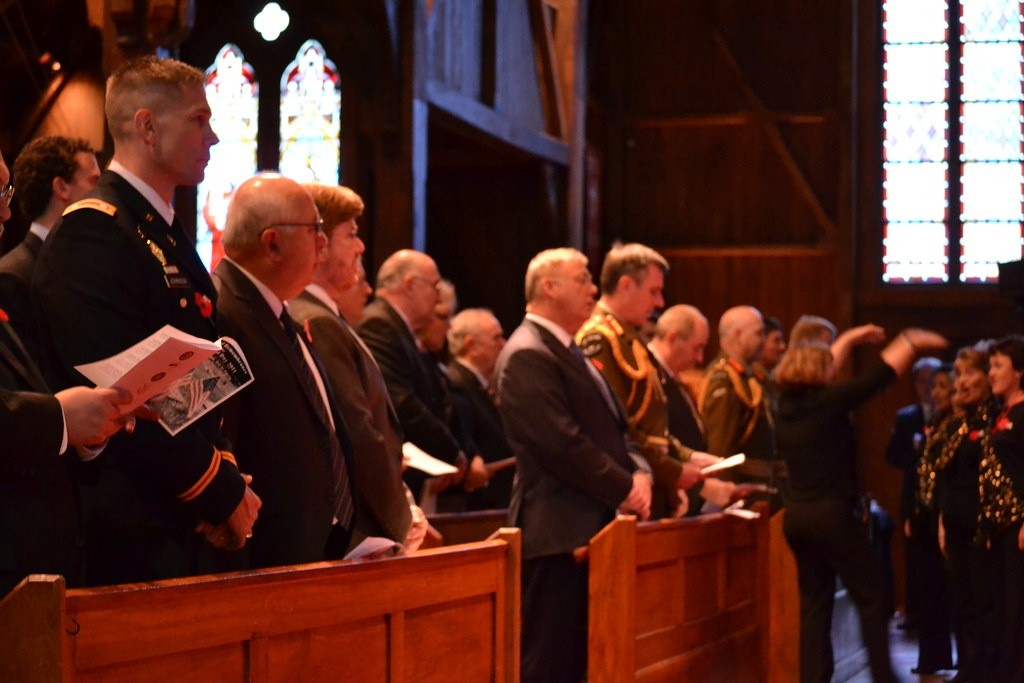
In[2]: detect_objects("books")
[75,325,256,439]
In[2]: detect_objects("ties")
[279,304,354,530]
[171,213,197,268]
[570,339,584,366]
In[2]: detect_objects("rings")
[219,536,225,542]
[246,533,252,538]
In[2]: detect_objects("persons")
[1,56,1024,683]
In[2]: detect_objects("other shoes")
[911,656,953,673]
[942,671,968,683]
[897,618,920,630]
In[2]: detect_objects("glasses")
[258,217,324,237]
[431,312,449,320]
[0,185,15,208]
